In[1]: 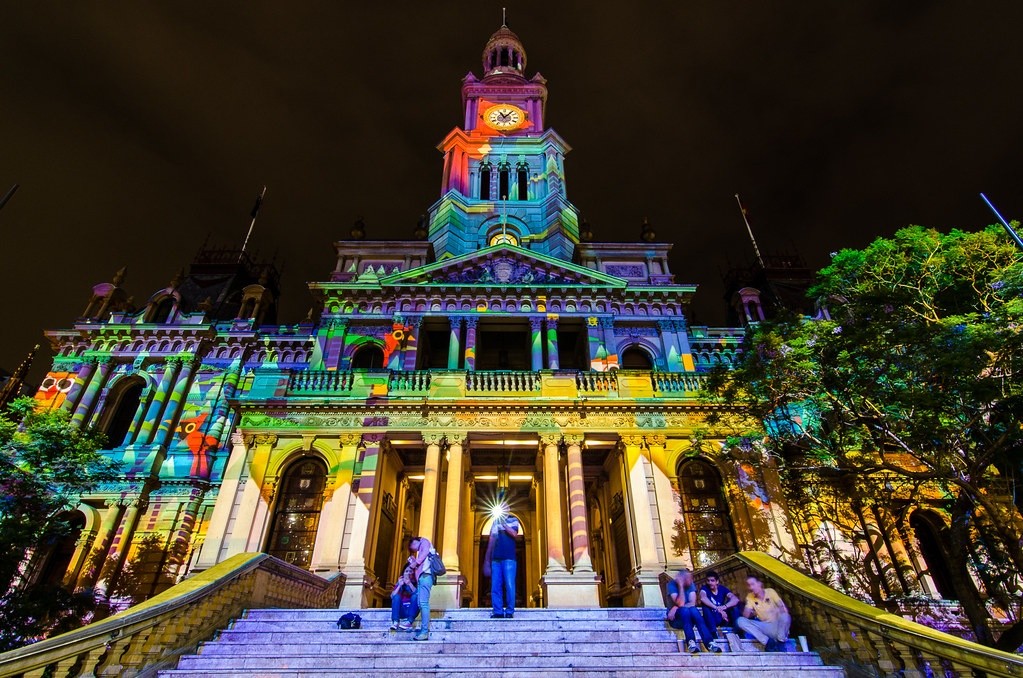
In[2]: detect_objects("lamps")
[497,434,512,488]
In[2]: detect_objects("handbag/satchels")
[420,538,446,576]
[336,612,362,629]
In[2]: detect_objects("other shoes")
[708,641,722,652]
[413,634,428,640]
[506,613,512,617]
[491,614,504,618]
[766,644,776,651]
[778,645,787,651]
[688,640,700,652]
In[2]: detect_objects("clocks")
[490,108,519,127]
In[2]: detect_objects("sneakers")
[399,621,413,629]
[390,623,398,630]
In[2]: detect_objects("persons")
[665,568,721,654]
[699,571,747,639]
[389,562,419,630]
[737,577,790,652]
[409,537,436,640]
[483,501,518,618]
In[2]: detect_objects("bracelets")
[724,604,727,609]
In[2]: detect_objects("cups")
[722,630,728,639]
[677,639,685,653]
[798,635,809,653]
